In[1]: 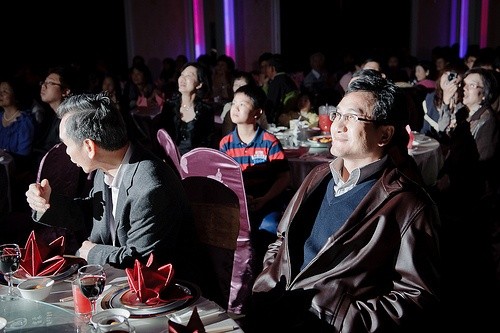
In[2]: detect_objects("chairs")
[157,129,255,321]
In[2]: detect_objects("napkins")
[125,252,195,306]
[2,230,88,277]
[168,310,205,333]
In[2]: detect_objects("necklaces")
[181,103,191,111]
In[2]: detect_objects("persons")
[0,49,500,333]
[236,75,440,333]
[26,90,197,288]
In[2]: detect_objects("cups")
[91,315,134,333]
[407,133,414,148]
[318,106,337,132]
[71,278,94,317]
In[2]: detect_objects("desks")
[275,131,442,191]
[0,268,244,333]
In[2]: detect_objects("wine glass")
[78,264,107,324]
[0,244,22,301]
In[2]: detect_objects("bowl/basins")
[0,317,8,333]
[17,279,54,300]
[90,307,130,331]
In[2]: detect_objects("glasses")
[463,82,484,90]
[39,80,63,88]
[329,111,375,126]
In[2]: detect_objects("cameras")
[448,72,457,81]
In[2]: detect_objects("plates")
[10,254,93,285]
[160,328,168,333]
[268,121,431,158]
[101,283,199,318]
